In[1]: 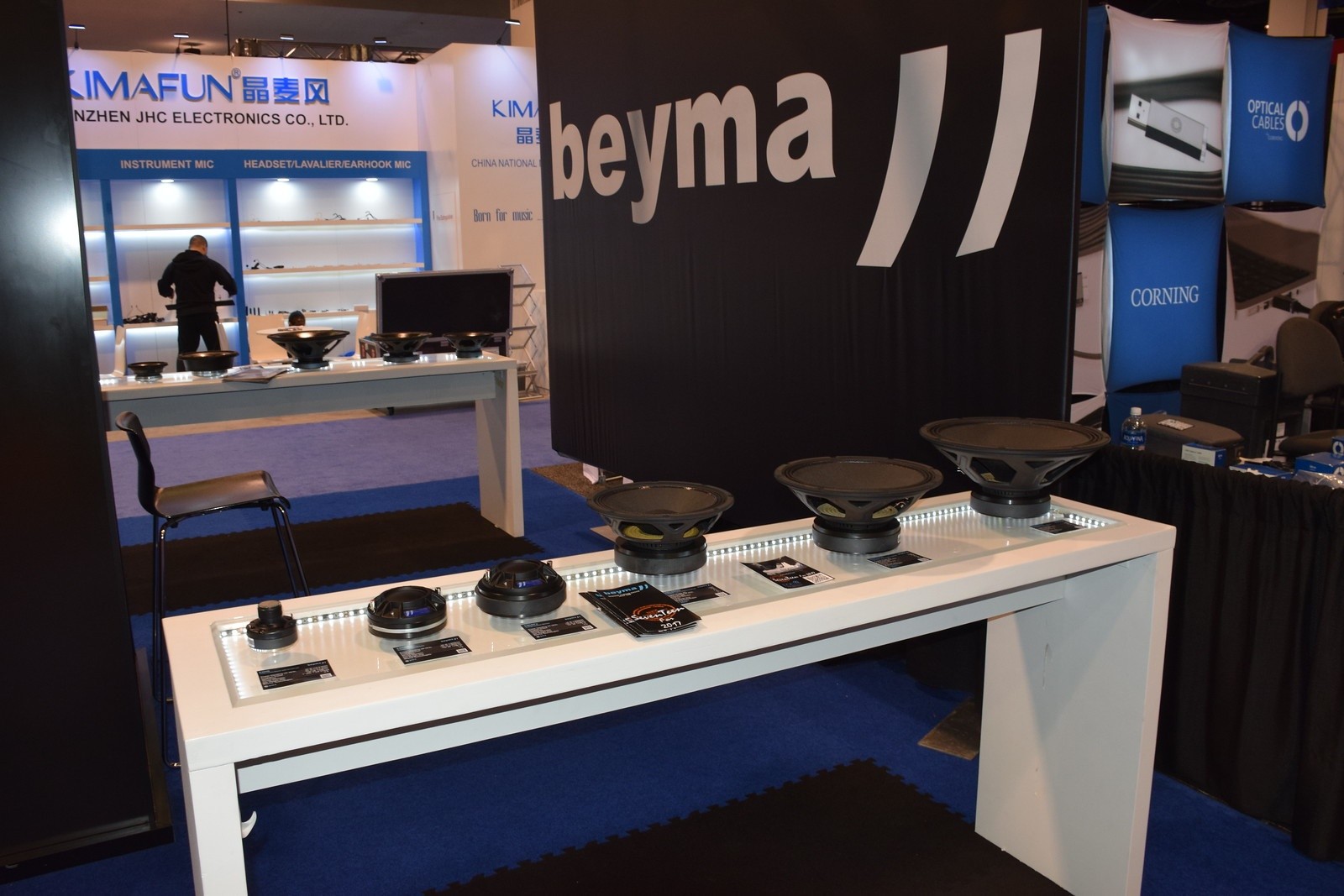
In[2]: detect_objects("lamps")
[173,31,189,53]
[279,33,295,56]
[68,24,86,50]
[498,18,520,45]
[372,37,387,62]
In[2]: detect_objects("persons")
[285,310,306,360]
[157,235,238,373]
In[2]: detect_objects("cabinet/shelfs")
[81,214,426,330]
[500,263,543,404]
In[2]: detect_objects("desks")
[161,489,1178,896]
[100,352,526,539]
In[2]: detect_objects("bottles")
[1121,407,1147,450]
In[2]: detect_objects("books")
[221,368,288,384]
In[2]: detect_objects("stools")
[114,410,311,771]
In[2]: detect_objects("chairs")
[244,314,292,369]
[351,311,377,359]
[100,326,127,378]
[1273,316,1344,454]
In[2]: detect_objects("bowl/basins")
[773,456,942,538]
[178,350,239,370]
[919,416,1111,504]
[588,480,735,558]
[127,362,167,375]
[267,329,350,362]
[362,332,432,356]
[443,330,493,351]
